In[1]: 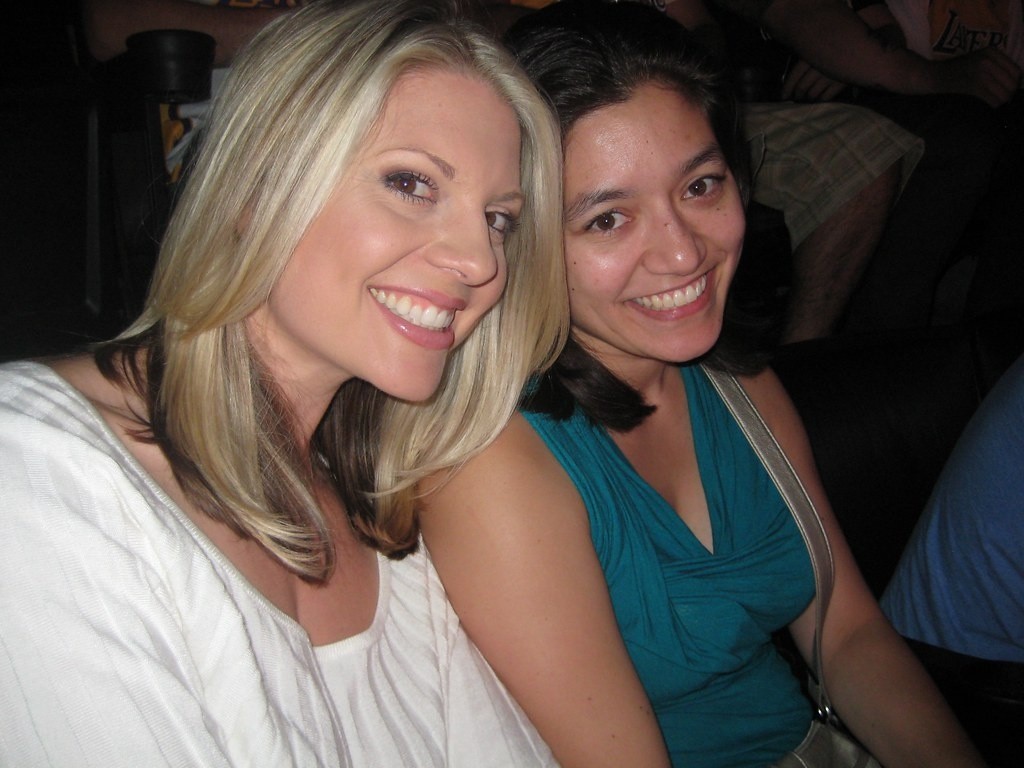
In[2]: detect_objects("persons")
[843,0,1021,335]
[0,0,570,768]
[877,355,1023,768]
[706,0,1023,344]
[626,0,773,104]
[415,0,988,768]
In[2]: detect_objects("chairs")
[728,87,1006,340]
[768,311,1023,597]
[35,1,215,345]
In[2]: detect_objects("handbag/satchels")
[777,722,880,768]
